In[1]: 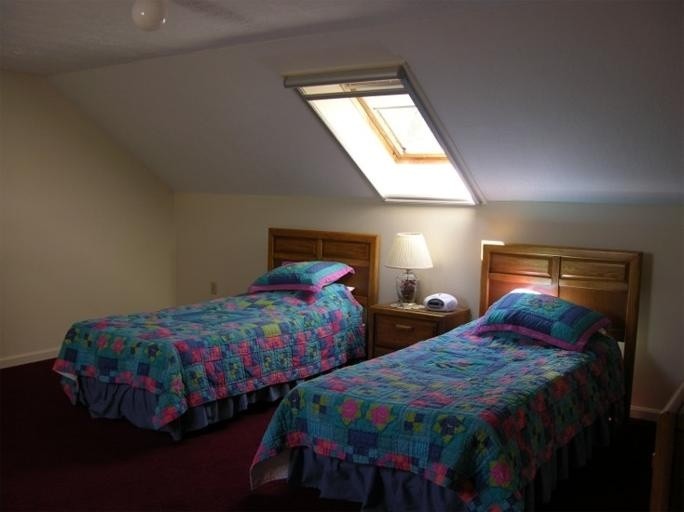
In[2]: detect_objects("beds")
[247,245,643,508]
[53,227,380,445]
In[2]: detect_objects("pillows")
[250,260,357,293]
[473,289,610,352]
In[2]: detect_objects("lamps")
[130,0,169,30]
[384,232,436,304]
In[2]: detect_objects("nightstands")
[368,303,470,358]
